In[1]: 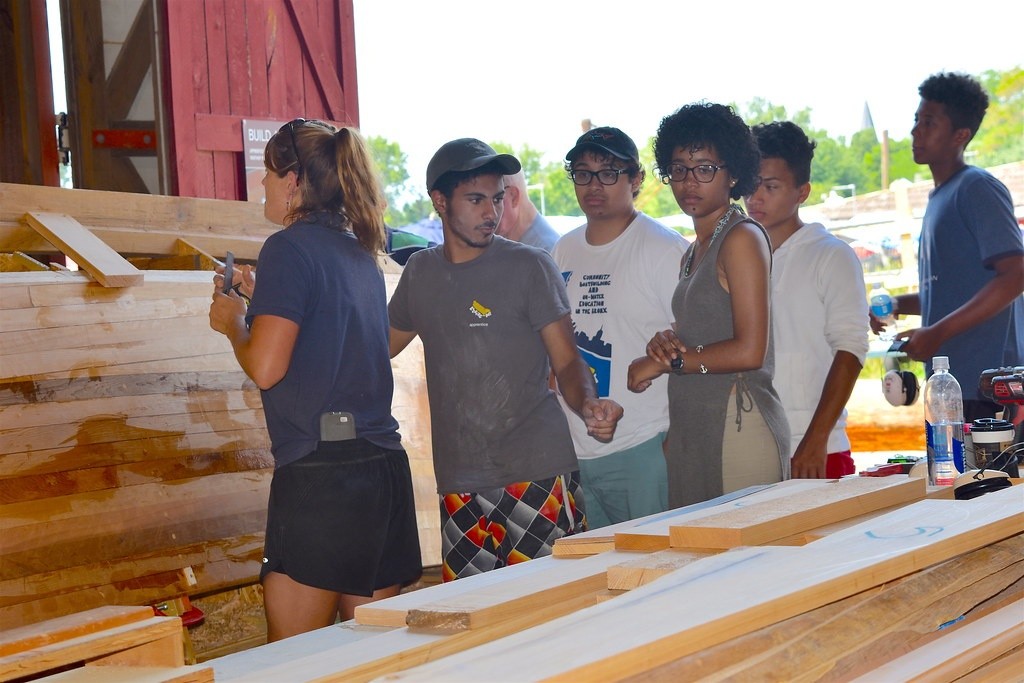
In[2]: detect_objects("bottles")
[925,357,965,494]
[964,423,976,471]
[869,283,898,341]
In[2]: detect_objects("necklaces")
[686,205,736,278]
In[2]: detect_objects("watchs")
[670,347,685,375]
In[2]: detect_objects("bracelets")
[696,345,707,374]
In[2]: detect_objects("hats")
[426,138,521,196]
[565,126,639,168]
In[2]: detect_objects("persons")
[388,137,625,585]
[868,67,1024,477]
[208,120,424,645]
[495,170,561,254]
[628,99,792,509]
[742,120,869,478]
[550,126,691,532]
[382,224,437,266]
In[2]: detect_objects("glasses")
[571,168,631,186]
[665,162,726,183]
[279,117,320,187]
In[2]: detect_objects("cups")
[970,417,1015,470]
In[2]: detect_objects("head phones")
[880,340,921,407]
